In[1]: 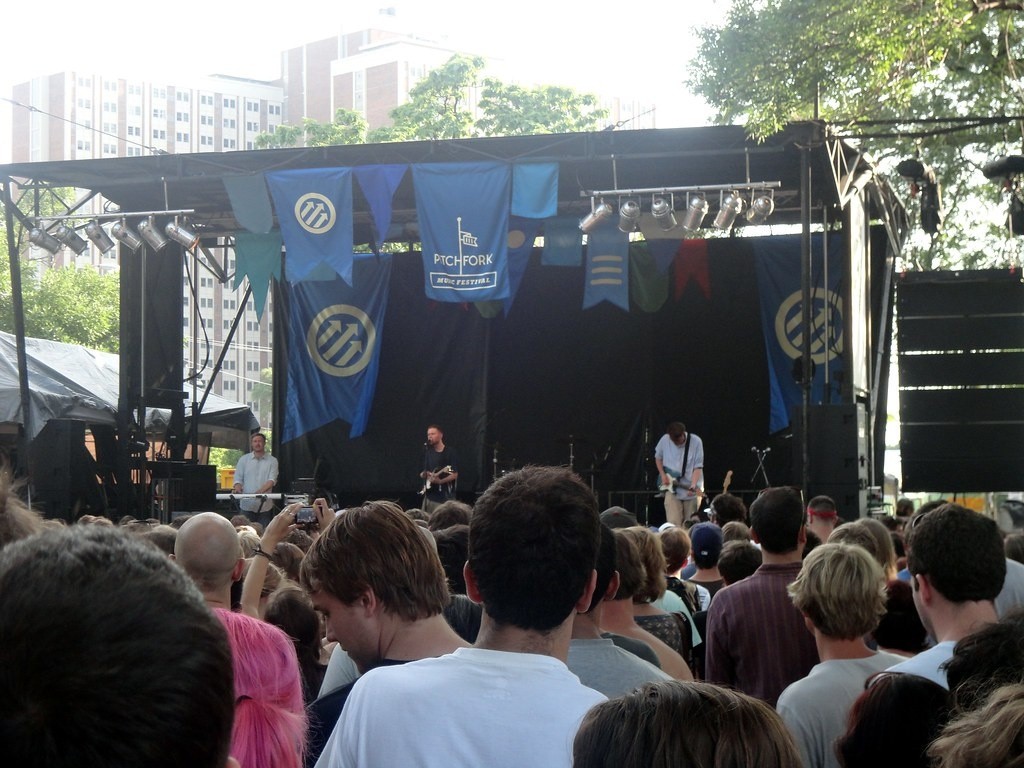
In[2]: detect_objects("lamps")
[896,159,938,190]
[575,179,785,235]
[26,206,209,256]
[983,151,1023,182]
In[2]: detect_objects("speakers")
[793,402,867,522]
[30,418,86,504]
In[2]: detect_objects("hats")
[703,500,721,519]
[687,521,724,556]
[599,507,640,534]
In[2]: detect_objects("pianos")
[216,492,288,522]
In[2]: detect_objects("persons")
[923,675,1024,768]
[864,506,1006,690]
[310,468,611,768]
[0,522,235,768]
[704,489,824,714]
[0,470,1024,682]
[233,433,280,525]
[575,681,805,768]
[210,604,306,768]
[654,421,706,529]
[305,500,471,768]
[775,544,913,767]
[830,672,953,768]
[418,425,459,513]
[946,601,1024,712]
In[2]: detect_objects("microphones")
[763,447,771,453]
[424,438,431,445]
[751,447,759,453]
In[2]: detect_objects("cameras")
[294,505,323,524]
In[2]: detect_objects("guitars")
[656,471,708,499]
[415,465,454,496]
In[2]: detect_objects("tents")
[0,330,260,453]
